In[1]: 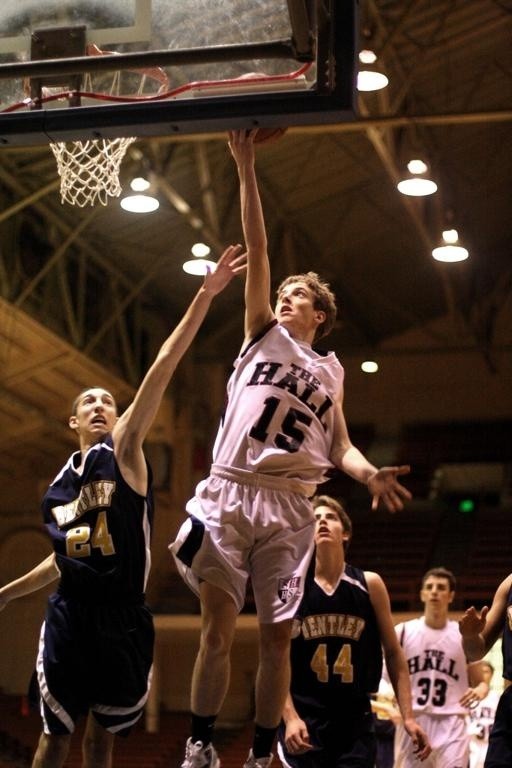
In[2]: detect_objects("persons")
[276,493,435,768]
[459,573,511,768]
[166,128,411,768]
[465,658,500,768]
[378,564,498,768]
[0,242,250,768]
[367,677,399,768]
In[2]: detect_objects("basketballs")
[228,72,289,146]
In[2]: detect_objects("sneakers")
[180,738,219,767]
[246,750,274,768]
